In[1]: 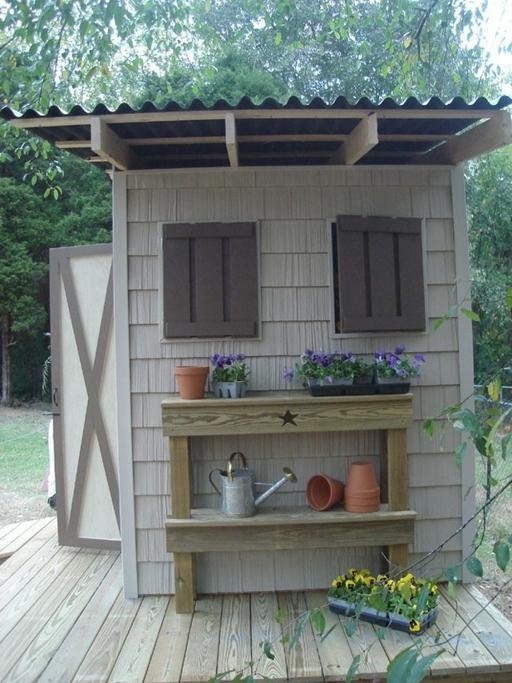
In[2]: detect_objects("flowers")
[327,567,437,632]
[208,352,251,383]
[283,348,374,391]
[373,345,427,380]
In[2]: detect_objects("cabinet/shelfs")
[161,392,418,615]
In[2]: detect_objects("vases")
[365,378,411,395]
[344,463,379,512]
[306,473,345,511]
[327,597,438,636]
[306,377,372,396]
[212,382,247,399]
[173,366,209,400]
